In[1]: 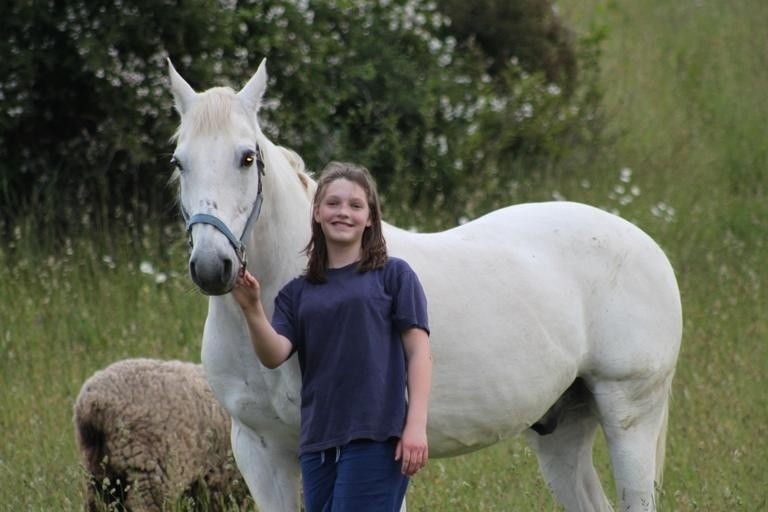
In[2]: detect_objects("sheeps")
[74,359,256,512]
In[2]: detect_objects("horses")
[166,57,682,512]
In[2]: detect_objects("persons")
[233,161,431,512]
